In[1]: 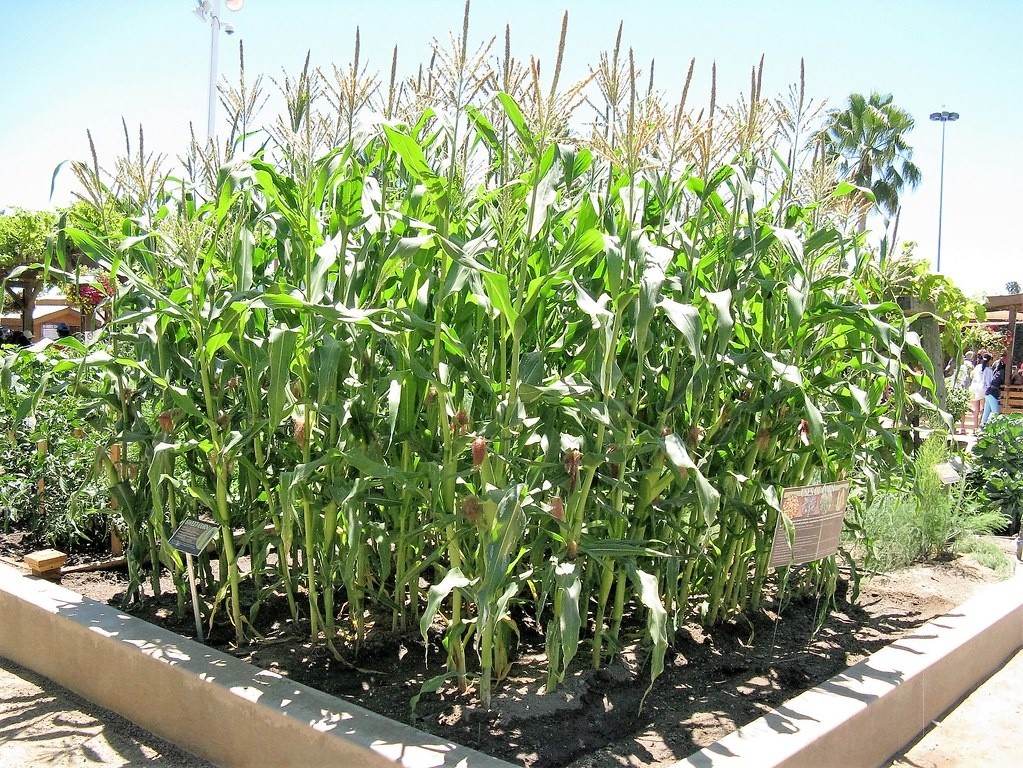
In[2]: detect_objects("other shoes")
[961,429,966,434]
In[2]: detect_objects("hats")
[1001,358,1006,364]
[23,330,36,338]
[978,350,987,358]
[55,323,69,330]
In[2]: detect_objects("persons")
[945,349,1023,436]
[53,323,71,341]
